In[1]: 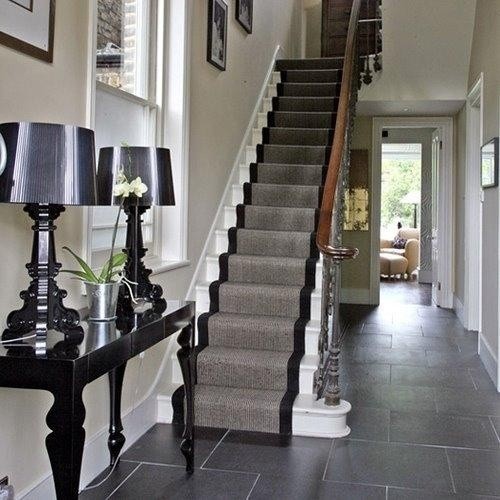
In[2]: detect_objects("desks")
[1,298,199,500]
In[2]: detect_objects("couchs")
[378,251,408,281]
[380,238,420,279]
[381,227,420,248]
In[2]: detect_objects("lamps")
[93,145,176,321]
[0,117,101,357]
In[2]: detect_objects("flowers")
[57,174,133,287]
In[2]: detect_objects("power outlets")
[0,475,16,500]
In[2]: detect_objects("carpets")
[167,53,362,441]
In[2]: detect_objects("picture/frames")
[478,136,499,189]
[205,0,229,71]
[1,0,56,64]
[234,0,254,34]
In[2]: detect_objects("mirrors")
[343,185,371,233]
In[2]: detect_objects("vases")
[80,280,121,325]
